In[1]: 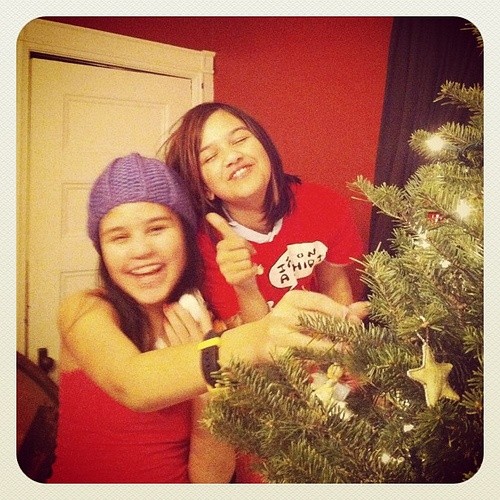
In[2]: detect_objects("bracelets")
[200,325,228,392]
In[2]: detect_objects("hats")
[87,151,197,256]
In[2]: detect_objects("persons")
[157,102,363,483]
[43,153,364,482]
